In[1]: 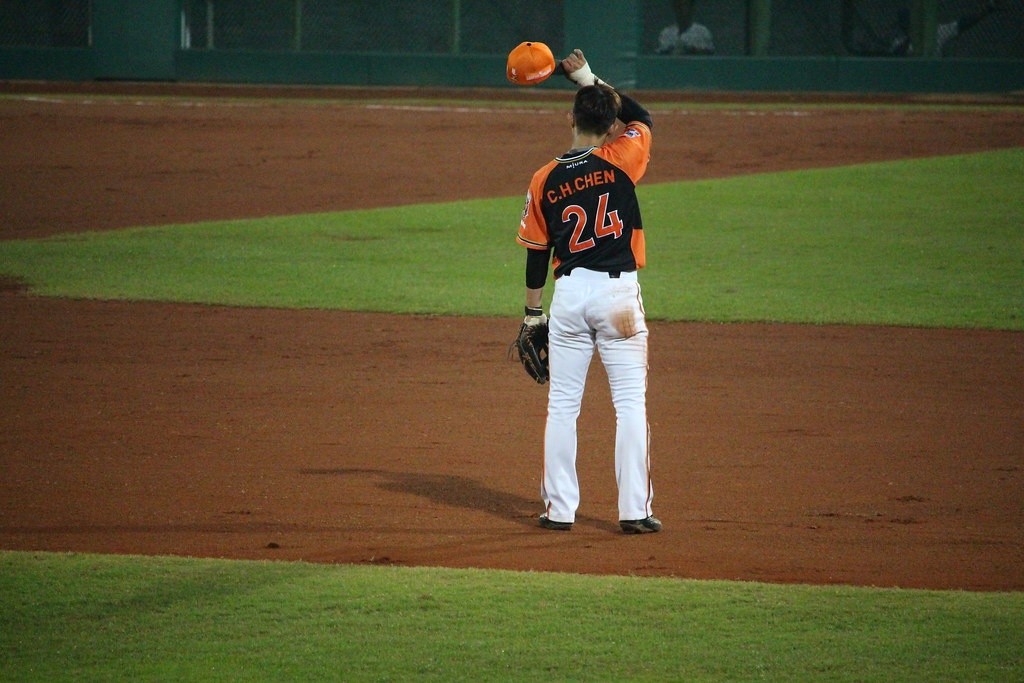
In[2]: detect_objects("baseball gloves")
[515,307,555,383]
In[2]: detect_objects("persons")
[515,48,662,533]
[658,0,715,56]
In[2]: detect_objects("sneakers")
[539,515,572,531]
[619,517,662,534]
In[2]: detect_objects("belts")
[562,266,621,278]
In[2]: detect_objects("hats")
[505,41,564,87]
